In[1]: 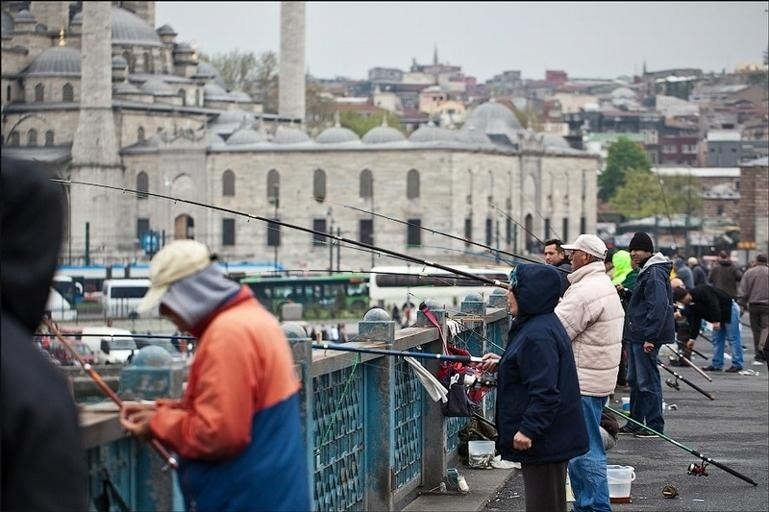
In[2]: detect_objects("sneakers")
[634,427,660,439]
[618,422,642,436]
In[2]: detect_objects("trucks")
[32,325,187,372]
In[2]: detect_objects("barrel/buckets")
[605,463,636,498]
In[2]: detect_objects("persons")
[613,232,677,439]
[475,263,590,510]
[0,160,91,512]
[549,234,625,510]
[528,235,768,378]
[118,239,311,512]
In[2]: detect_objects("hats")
[135,238,211,314]
[559,233,608,260]
[628,231,654,253]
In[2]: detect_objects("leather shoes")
[701,365,722,372]
[725,366,742,373]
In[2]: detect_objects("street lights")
[736,240,758,267]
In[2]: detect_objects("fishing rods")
[698,330,715,345]
[49,176,510,291]
[314,196,574,274]
[32,330,500,367]
[402,238,523,269]
[42,315,182,473]
[674,336,709,362]
[665,343,715,383]
[488,204,715,400]
[408,288,757,492]
[523,196,665,366]
